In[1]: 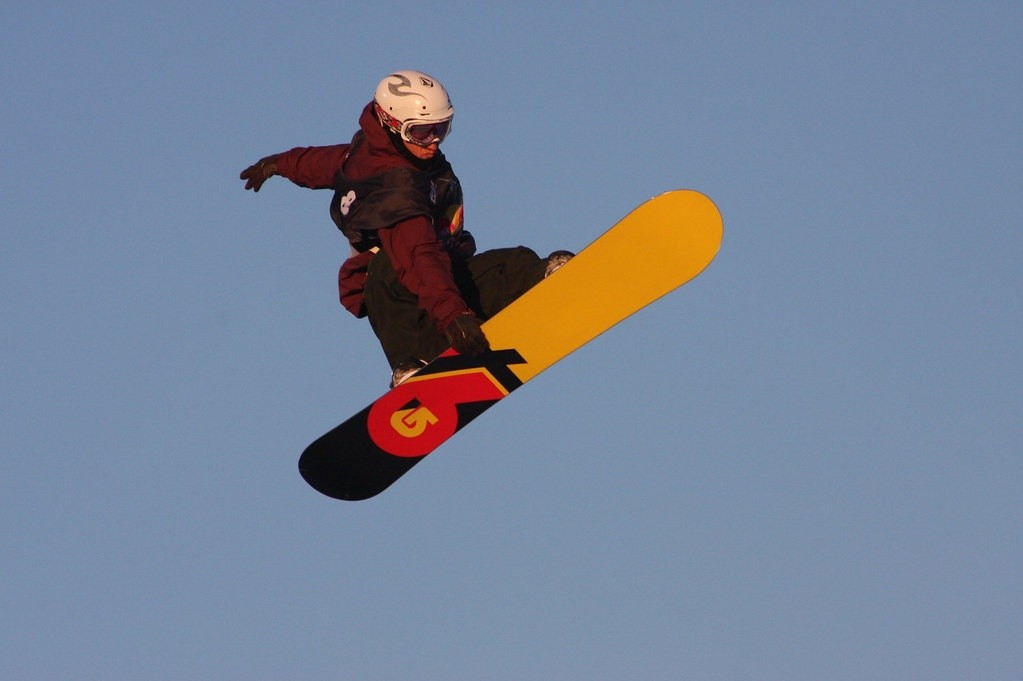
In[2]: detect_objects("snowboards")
[297,188,724,502]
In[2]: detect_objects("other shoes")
[391,361,421,388]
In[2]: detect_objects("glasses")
[408,120,448,143]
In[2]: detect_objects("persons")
[239,71,575,387]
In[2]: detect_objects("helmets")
[374,70,456,142]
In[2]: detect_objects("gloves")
[445,315,491,356]
[240,154,279,193]
